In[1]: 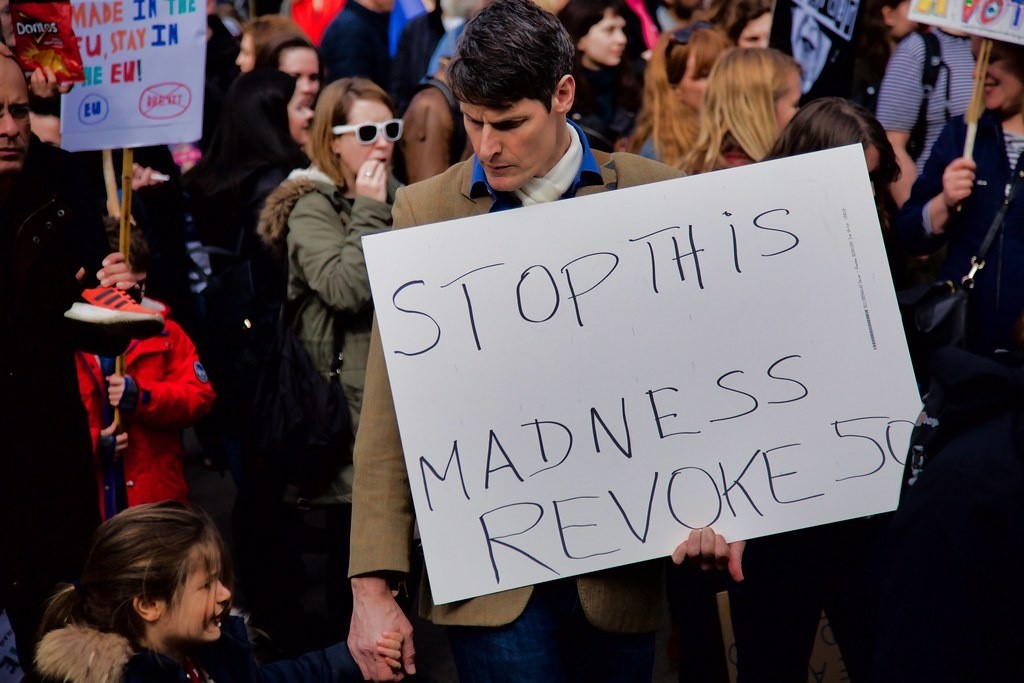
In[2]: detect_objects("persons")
[0,0,1024,683]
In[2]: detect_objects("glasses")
[332,118,405,146]
[665,18,715,59]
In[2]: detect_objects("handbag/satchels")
[906,279,982,345]
[241,293,353,472]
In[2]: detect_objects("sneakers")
[62,284,167,339]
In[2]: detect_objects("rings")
[364,172,374,178]
